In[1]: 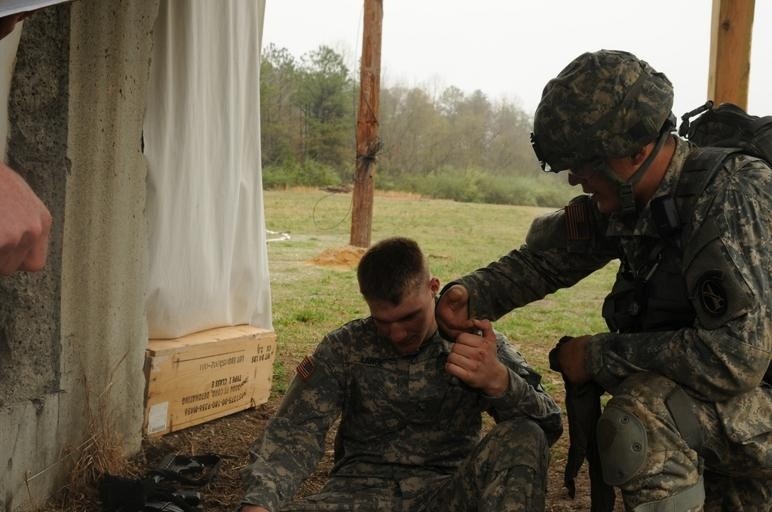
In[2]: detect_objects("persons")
[1,6,55,276]
[244,236,563,512]
[436,50,771,509]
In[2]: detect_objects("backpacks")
[675,101,772,247]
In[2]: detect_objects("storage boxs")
[143,320,281,443]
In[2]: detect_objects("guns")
[139,449,222,512]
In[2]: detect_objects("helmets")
[531,49,673,174]
